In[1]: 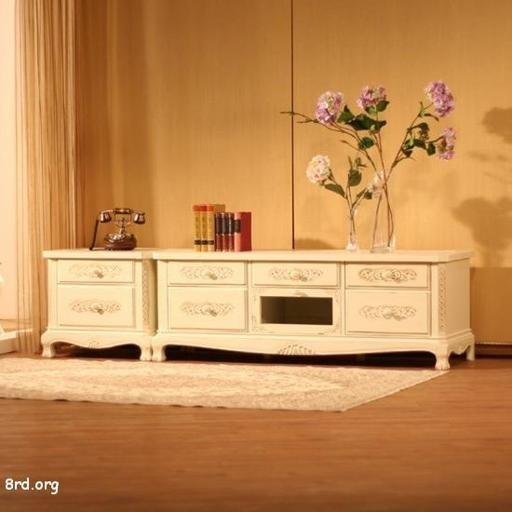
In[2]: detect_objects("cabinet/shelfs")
[39,246,478,374]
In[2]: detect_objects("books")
[191,202,252,252]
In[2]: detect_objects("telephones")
[99,208,145,250]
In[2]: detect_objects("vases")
[341,168,396,252]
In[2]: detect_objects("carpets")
[1,348,453,412]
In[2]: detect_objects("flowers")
[274,81,456,243]
